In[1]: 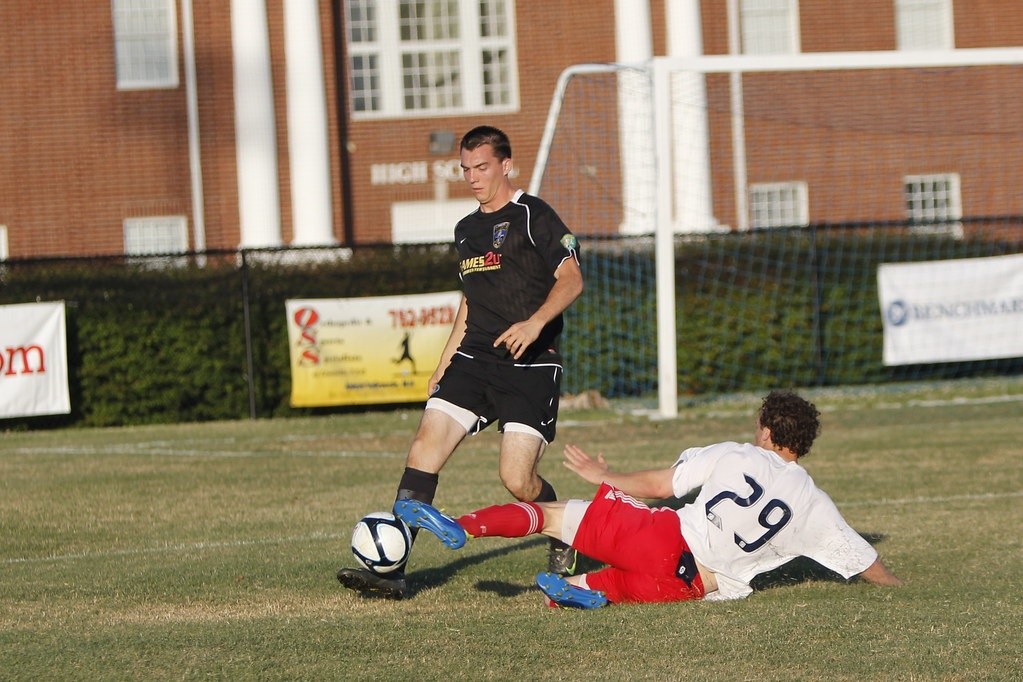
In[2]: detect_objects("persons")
[394,390,911,608]
[338,126,583,600]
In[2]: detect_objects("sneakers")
[544,538,580,577]
[338,566,406,597]
[393,497,474,550]
[535,571,608,609]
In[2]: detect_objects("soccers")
[351,510,413,574]
